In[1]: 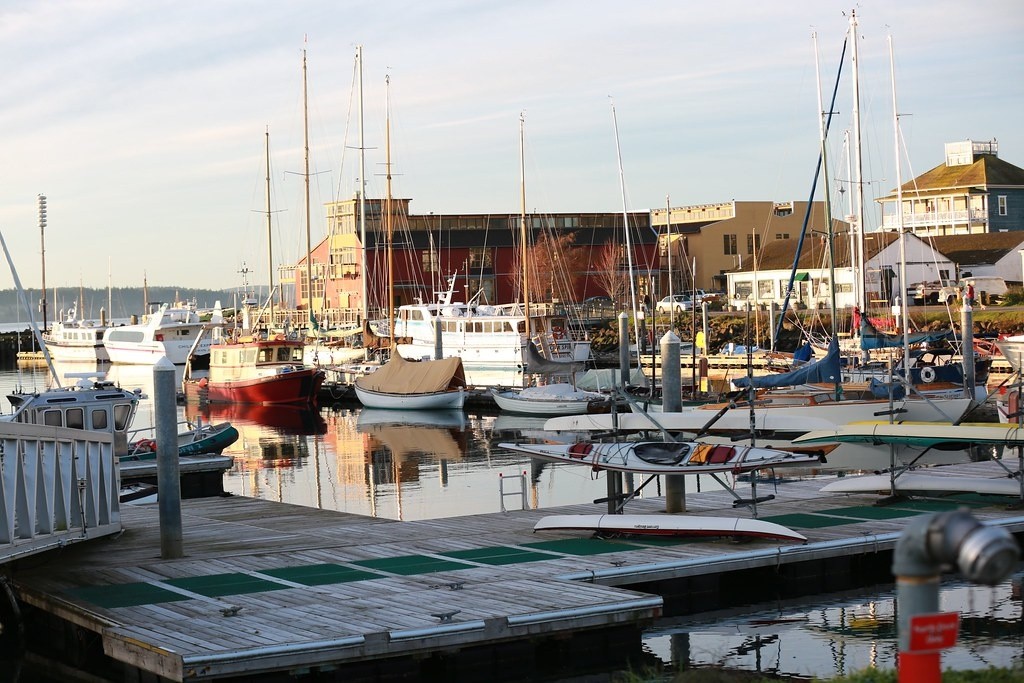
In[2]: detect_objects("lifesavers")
[920,367,936,383]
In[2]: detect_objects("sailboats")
[1,7,1024,533]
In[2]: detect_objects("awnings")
[882,269,897,279]
[794,272,808,281]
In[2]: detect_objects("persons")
[966,284,974,310]
[643,295,651,316]
